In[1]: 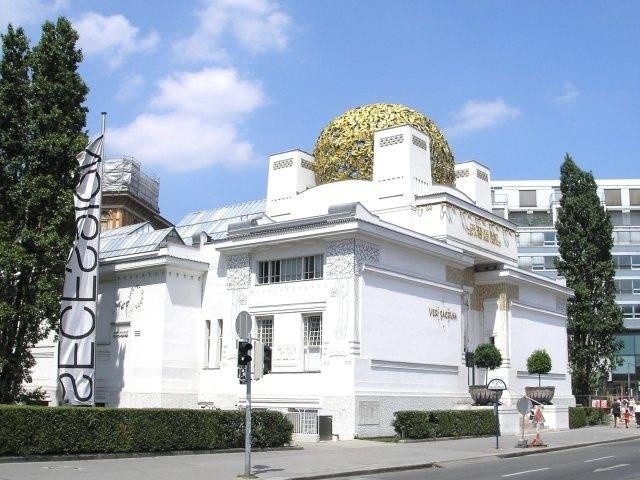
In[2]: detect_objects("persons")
[616,396,636,415]
[623,409,631,428]
[611,399,622,428]
[634,401,640,428]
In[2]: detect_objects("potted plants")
[469,343,504,405]
[524,348,555,404]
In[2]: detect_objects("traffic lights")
[236,340,252,369]
[254,341,271,380]
